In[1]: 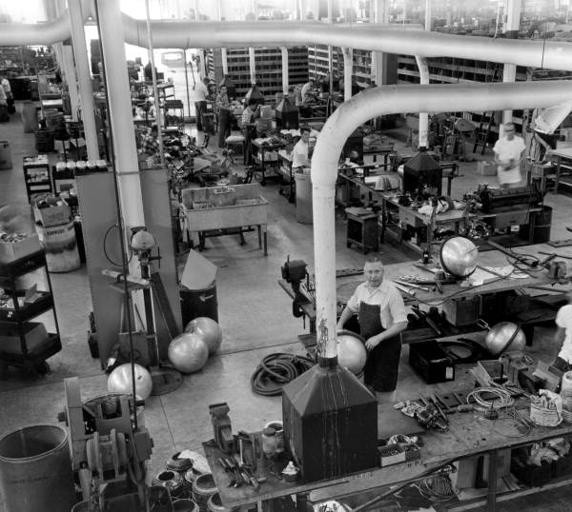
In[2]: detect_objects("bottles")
[262,427,276,454]
[269,423,285,454]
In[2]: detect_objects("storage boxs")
[35,193,70,227]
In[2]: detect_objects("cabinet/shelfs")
[24,165,52,204]
[0,248,62,367]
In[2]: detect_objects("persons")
[0,75,14,96]
[215,85,234,149]
[334,255,410,402]
[549,301,572,372]
[288,124,314,180]
[299,80,315,103]
[1,83,8,107]
[492,120,526,187]
[241,97,262,163]
[193,76,211,134]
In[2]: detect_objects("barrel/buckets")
[294,168,312,225]
[260,105,271,120]
[0,141,12,169]
[201,112,216,135]
[46,111,66,139]
[0,423,73,510]
[34,218,80,274]
[195,100,207,131]
